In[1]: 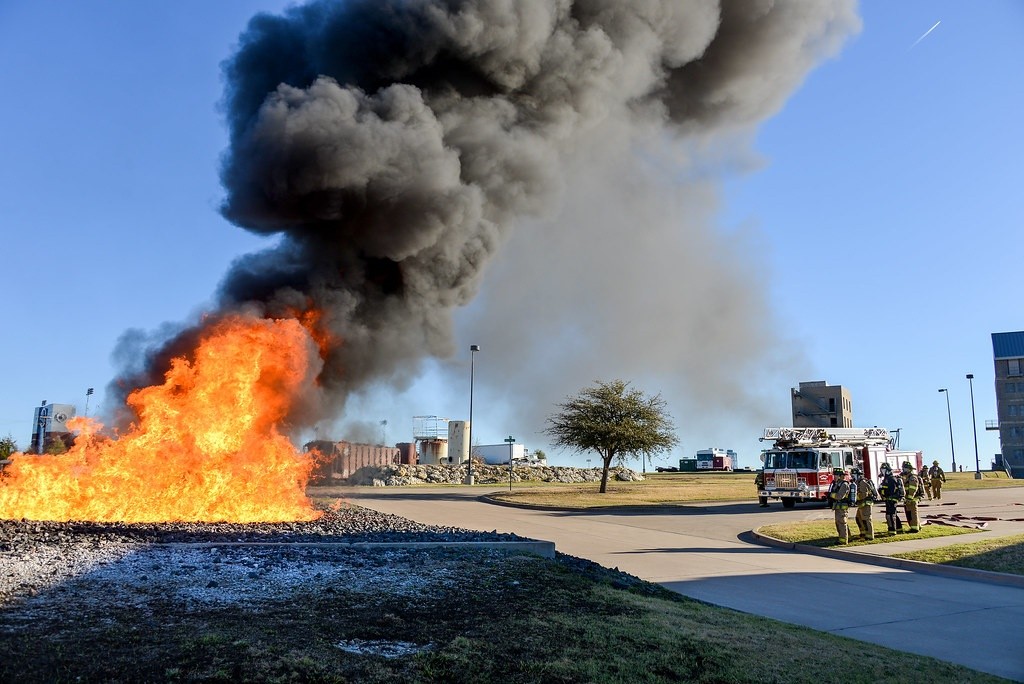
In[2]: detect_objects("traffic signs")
[504,438,515,442]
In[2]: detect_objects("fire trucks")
[757,424,924,509]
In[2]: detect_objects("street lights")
[468,343,480,475]
[965,373,981,478]
[84,388,94,416]
[939,388,957,472]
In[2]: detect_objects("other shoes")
[834,540,845,545]
[888,533,895,536]
[897,530,903,534]
[859,533,865,537]
[864,537,873,540]
[910,529,919,533]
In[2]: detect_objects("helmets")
[923,465,928,469]
[933,461,939,464]
[832,468,845,475]
[881,463,891,469]
[902,461,912,468]
[850,468,864,474]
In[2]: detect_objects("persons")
[929,461,946,499]
[897,461,925,532]
[851,468,877,541]
[878,463,903,535]
[918,465,932,501]
[826,467,852,544]
[754,472,770,508]
